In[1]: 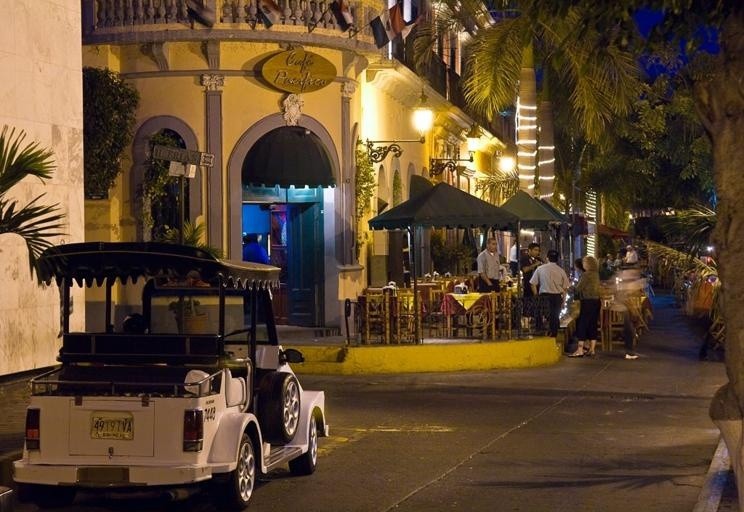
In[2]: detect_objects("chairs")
[362,266,647,355]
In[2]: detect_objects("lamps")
[432,119,483,173]
[366,86,435,145]
[476,145,518,183]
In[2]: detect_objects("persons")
[476,238,501,293]
[566,245,642,360]
[519,242,543,339]
[529,248,569,336]
[104,313,168,366]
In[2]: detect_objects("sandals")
[568,351,595,357]
